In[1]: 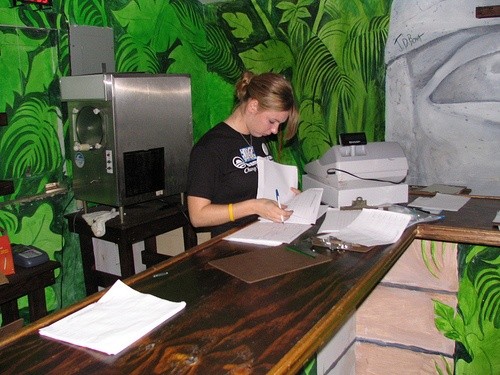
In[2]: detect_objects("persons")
[188,72,305,237]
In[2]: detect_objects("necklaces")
[234,125,256,155]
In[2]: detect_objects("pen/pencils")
[276,189,284,224]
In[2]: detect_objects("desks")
[65,195,197,296]
[0,191,500,375]
[0,261,62,325]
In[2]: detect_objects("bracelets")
[227,203,236,223]
